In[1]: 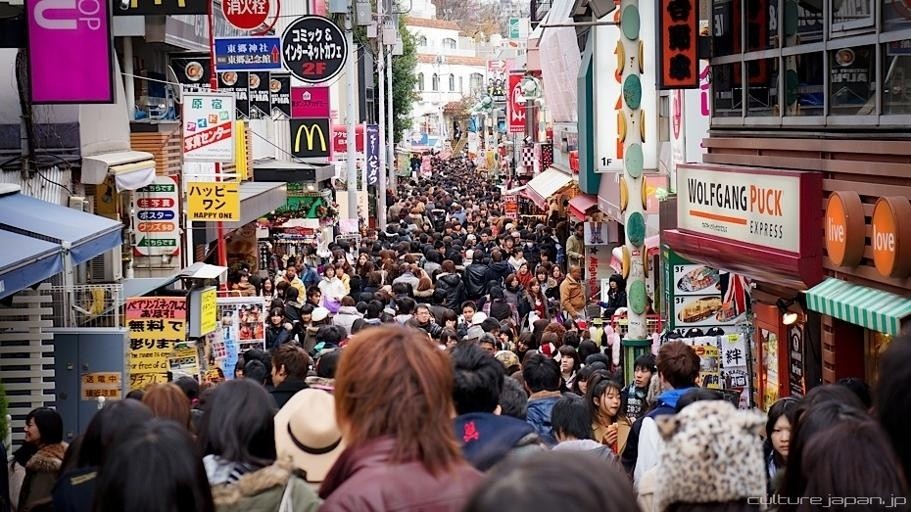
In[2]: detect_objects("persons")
[493,350,520,376]
[18,407,71,512]
[471,449,639,512]
[235,359,247,379]
[586,353,609,371]
[809,384,863,411]
[126,389,144,401]
[550,397,630,488]
[554,346,580,392]
[579,340,598,368]
[229,154,567,354]
[622,354,655,425]
[245,359,268,385]
[498,377,529,418]
[760,396,808,512]
[875,330,908,485]
[523,355,565,449]
[591,273,627,320]
[50,399,156,511]
[779,400,873,509]
[559,265,587,325]
[176,376,200,410]
[800,421,911,509]
[143,383,198,444]
[6,442,40,512]
[588,369,631,454]
[620,340,703,479]
[574,367,593,396]
[563,318,579,354]
[621,295,656,334]
[197,377,324,512]
[565,221,585,281]
[94,416,216,512]
[443,345,542,470]
[839,377,877,418]
[57,432,86,478]
[266,344,313,413]
[273,386,351,494]
[635,389,770,512]
[312,325,484,512]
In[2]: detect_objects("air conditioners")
[89,244,122,283]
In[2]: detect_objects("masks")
[526,242,533,248]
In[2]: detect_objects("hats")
[312,306,330,322]
[535,342,563,372]
[534,223,545,230]
[653,399,769,512]
[471,311,488,325]
[466,234,477,240]
[466,325,484,340]
[274,387,352,481]
[504,223,513,231]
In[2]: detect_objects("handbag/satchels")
[323,294,341,313]
[518,297,532,317]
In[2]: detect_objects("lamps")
[776,296,800,325]
